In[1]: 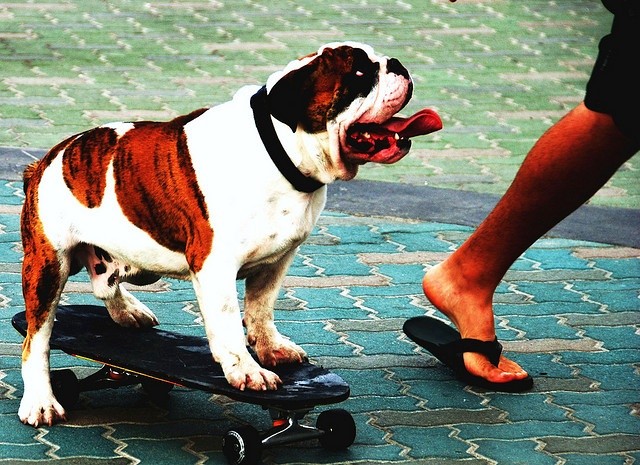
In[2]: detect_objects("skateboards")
[11,305,356,461]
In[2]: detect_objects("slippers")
[402,316,533,391]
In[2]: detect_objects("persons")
[402,1,640,393]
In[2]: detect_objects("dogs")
[18,41,443,428]
